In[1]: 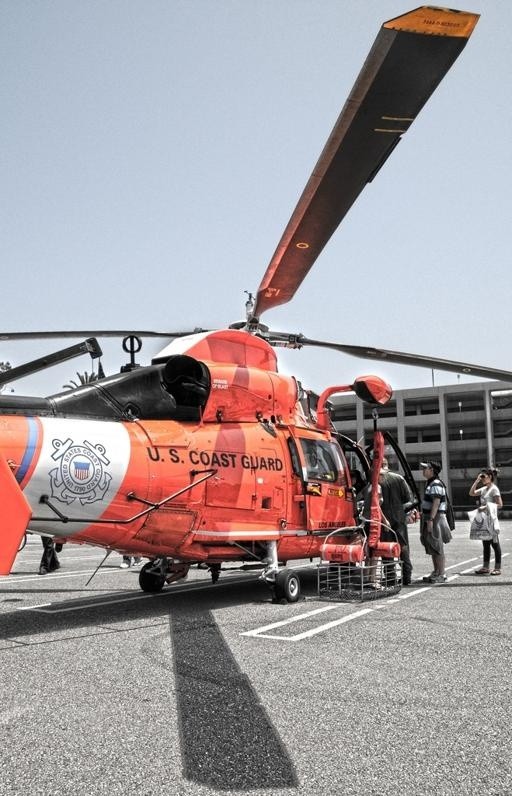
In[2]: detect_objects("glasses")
[480,475,488,479]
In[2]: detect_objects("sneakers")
[475,568,501,576]
[422,572,448,584]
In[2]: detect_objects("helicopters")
[0,4,512,604]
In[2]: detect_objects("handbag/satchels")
[445,502,455,531]
[468,509,492,541]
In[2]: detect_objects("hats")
[420,461,441,473]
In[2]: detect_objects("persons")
[38,535,61,575]
[360,470,391,540]
[357,458,414,586]
[418,460,453,583]
[470,468,503,575]
[120,555,143,569]
